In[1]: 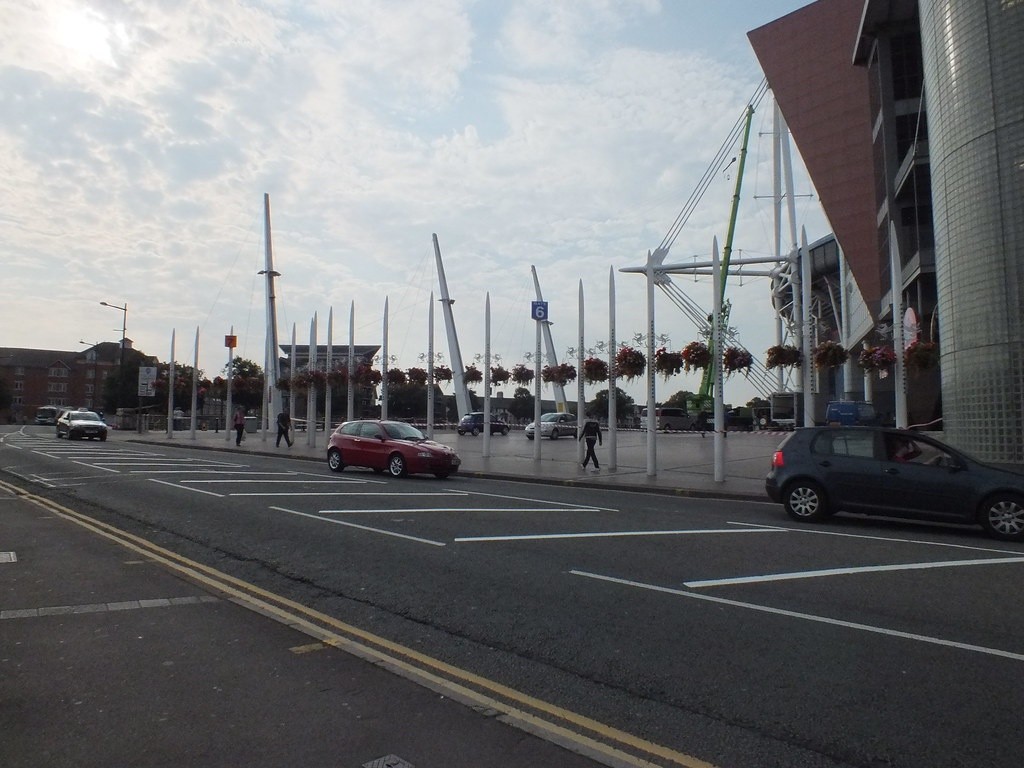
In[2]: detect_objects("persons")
[274,408,295,448]
[233,406,246,446]
[579,411,603,472]
[889,437,923,463]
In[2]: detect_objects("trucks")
[826,400,878,428]
[769,391,804,431]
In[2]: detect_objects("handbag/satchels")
[241,428,247,441]
[288,428,294,443]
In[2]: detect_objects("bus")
[34,406,58,424]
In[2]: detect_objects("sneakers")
[580,462,585,471]
[589,467,601,473]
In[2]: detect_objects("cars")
[457,412,512,437]
[56,411,107,442]
[525,412,578,440]
[765,423,1024,540]
[325,419,462,480]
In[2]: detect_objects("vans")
[641,407,697,432]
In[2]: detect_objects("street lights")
[99,300,127,409]
[79,341,98,410]
